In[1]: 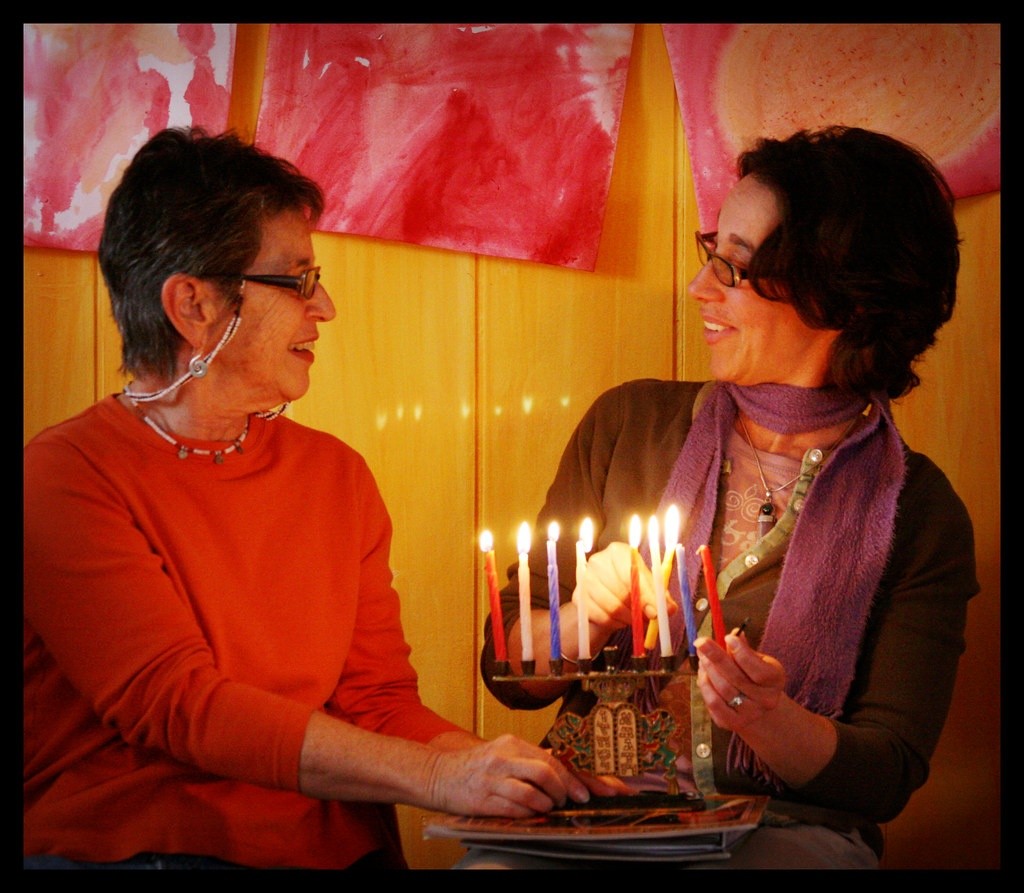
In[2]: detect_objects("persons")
[478,117,982,870]
[22,124,590,872]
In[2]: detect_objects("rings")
[726,691,748,709]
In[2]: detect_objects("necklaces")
[731,396,866,548]
[118,385,256,466]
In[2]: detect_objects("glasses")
[196,266,321,300]
[695,231,768,286]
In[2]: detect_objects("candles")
[647,514,674,658]
[546,518,561,661]
[675,542,699,658]
[574,516,594,660]
[626,513,647,659]
[643,503,682,650]
[516,520,534,662]
[695,543,728,653]
[478,530,508,662]
